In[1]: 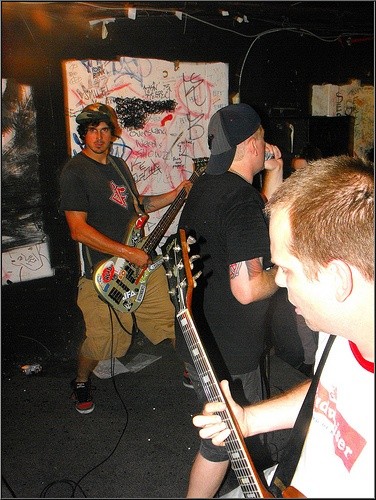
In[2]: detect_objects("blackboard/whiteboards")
[59,55,234,255]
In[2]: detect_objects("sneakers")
[72,378,95,414]
[183,370,193,389]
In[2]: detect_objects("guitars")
[93,157,210,313]
[160,228,309,499]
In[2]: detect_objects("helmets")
[76,103,118,129]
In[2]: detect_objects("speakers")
[267,117,310,158]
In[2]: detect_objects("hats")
[206,103,260,176]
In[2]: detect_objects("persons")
[192,155,375,499]
[60,102,194,414]
[293,157,317,378]
[252,109,288,176]
[177,103,309,499]
[284,119,322,178]
